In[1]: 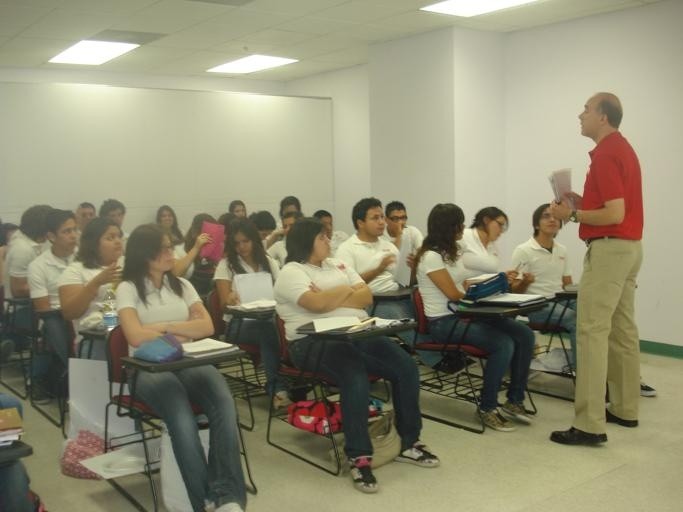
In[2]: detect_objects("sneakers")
[24,379,52,404]
[0,339,16,361]
[501,398,532,424]
[304,385,341,401]
[475,407,515,431]
[350,455,379,493]
[446,352,478,368]
[274,393,294,410]
[639,377,656,398]
[395,443,439,468]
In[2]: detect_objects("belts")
[585,236,617,247]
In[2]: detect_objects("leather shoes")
[605,408,638,428]
[432,359,467,373]
[550,425,607,445]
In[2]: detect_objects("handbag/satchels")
[457,272,509,321]
[329,408,401,470]
[287,398,342,435]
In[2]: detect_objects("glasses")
[496,219,505,232]
[389,215,407,222]
[161,242,174,252]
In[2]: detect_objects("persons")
[1,195,657,510]
[546,90,645,447]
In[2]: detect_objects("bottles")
[102,285,119,331]
